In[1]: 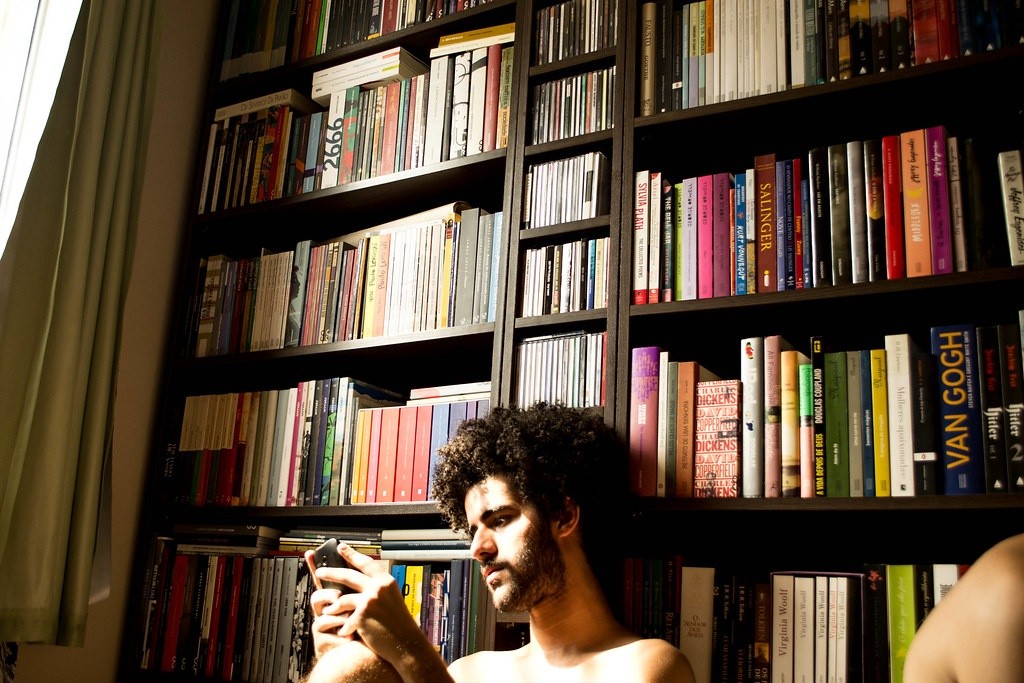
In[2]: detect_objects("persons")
[902,533,1024,683]
[303,401,696,683]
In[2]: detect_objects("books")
[632,126,1024,305]
[518,329,608,413]
[129,522,529,683]
[629,311,1024,500]
[188,1,516,362]
[530,0,618,67]
[157,374,492,506]
[522,151,611,229]
[621,540,972,683]
[528,66,616,145]
[635,0,1024,120]
[522,236,609,317]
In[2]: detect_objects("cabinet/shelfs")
[114,0,1024,683]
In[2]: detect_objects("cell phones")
[313,538,361,640]
